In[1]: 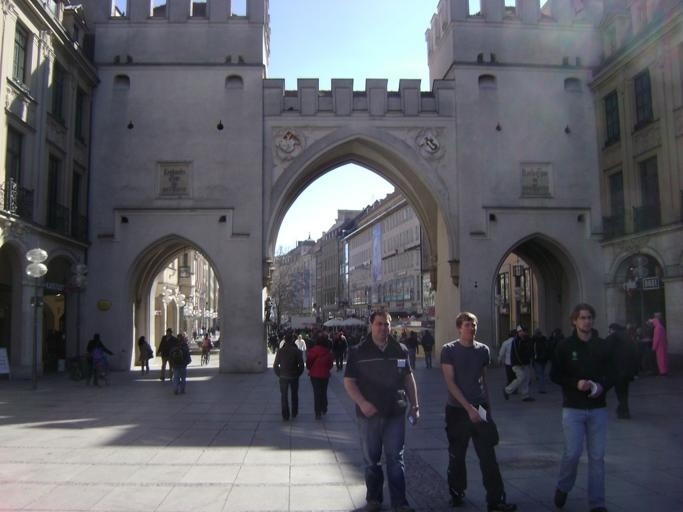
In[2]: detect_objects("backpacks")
[169,348,188,366]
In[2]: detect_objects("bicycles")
[200,344,214,366]
[85,353,112,387]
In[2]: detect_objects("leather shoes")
[522,397,536,402]
[502,388,510,401]
[366,498,380,512]
[392,500,414,512]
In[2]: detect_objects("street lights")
[26,250,46,391]
[168,292,185,339]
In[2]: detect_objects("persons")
[399,330,408,346]
[497,330,520,395]
[304,333,334,421]
[405,329,418,370]
[303,333,312,352]
[421,330,435,369]
[167,332,191,396]
[439,311,517,511]
[294,334,306,360]
[84,332,118,388]
[603,322,638,420]
[332,331,349,373]
[549,302,624,512]
[273,333,304,421]
[547,327,564,362]
[199,333,212,366]
[137,335,154,375]
[529,328,549,395]
[646,311,670,377]
[266,323,362,347]
[342,307,420,512]
[157,327,178,383]
[501,322,536,403]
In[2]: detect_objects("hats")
[516,323,527,332]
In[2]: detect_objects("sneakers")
[283,415,287,420]
[554,488,568,508]
[315,413,320,418]
[292,411,297,417]
[322,409,327,414]
[592,506,606,512]
[449,495,467,512]
[487,501,518,512]
